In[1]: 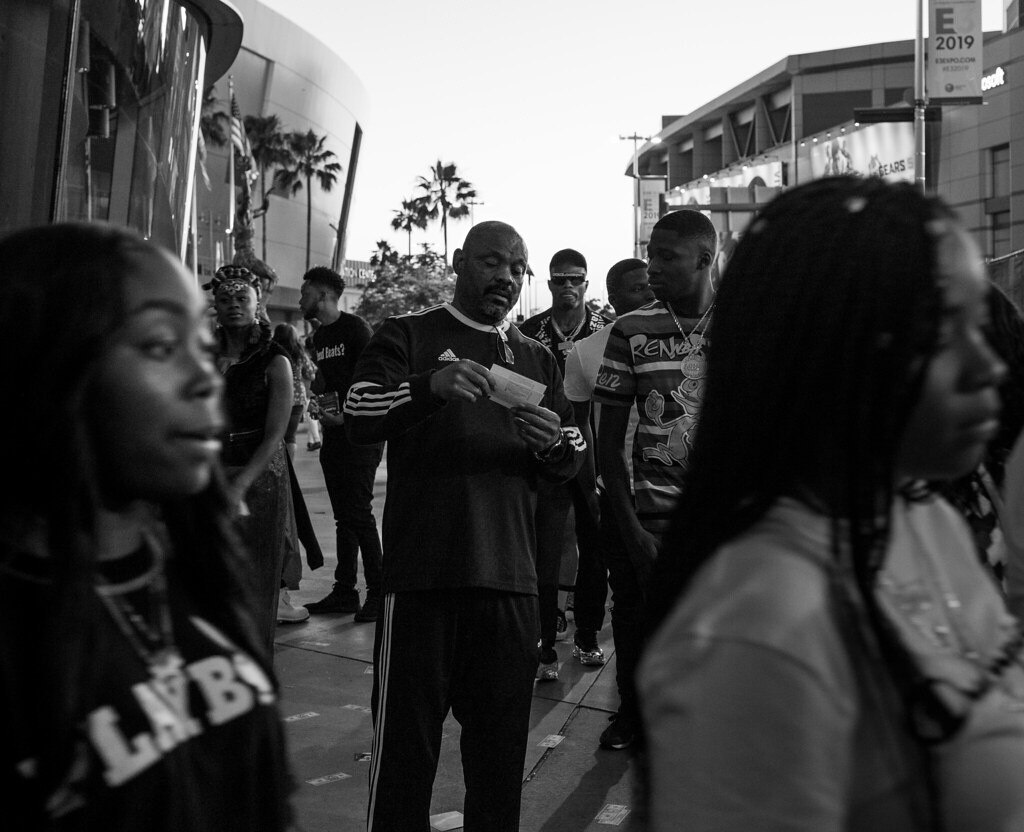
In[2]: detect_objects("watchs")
[534,427,564,463]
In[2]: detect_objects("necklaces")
[84,525,184,679]
[666,290,716,380]
[769,500,982,668]
[551,311,588,355]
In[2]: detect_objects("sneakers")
[565,591,578,622]
[556,609,568,641]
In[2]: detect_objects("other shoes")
[307,442,321,451]
[535,650,559,684]
[598,711,639,749]
[572,633,605,667]
[354,589,381,623]
[302,588,362,614]
[275,588,309,623]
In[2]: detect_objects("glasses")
[552,272,585,286]
[493,325,515,366]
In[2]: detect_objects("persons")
[514,249,657,681]
[0,220,298,832]
[634,173,1024,832]
[341,220,587,832]
[592,211,724,749]
[200,265,386,675]
[927,281,1024,623]
